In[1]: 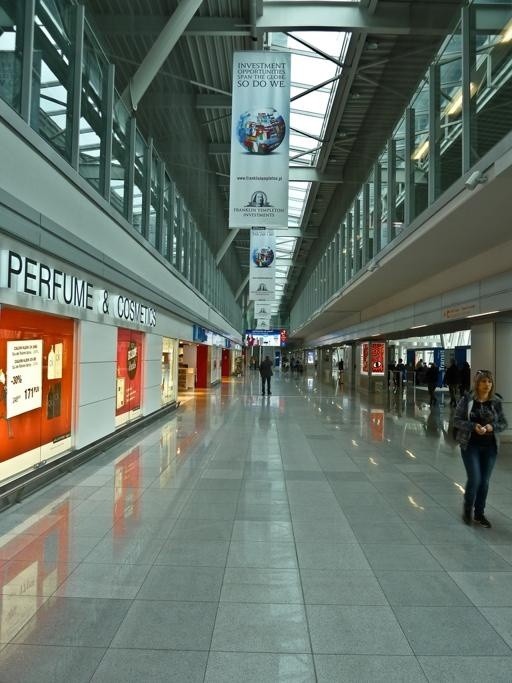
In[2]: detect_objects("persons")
[388,356,471,406]
[253,192,264,207]
[253,355,258,370]
[260,355,274,395]
[282,356,304,373]
[452,369,507,527]
[178,354,184,362]
[249,356,253,369]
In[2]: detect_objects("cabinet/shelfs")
[177,367,192,390]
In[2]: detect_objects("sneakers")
[474,514,490,527]
[463,503,472,525]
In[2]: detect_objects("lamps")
[442,80,478,116]
[497,16,511,45]
[409,138,429,162]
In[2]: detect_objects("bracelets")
[483,425,488,431]
